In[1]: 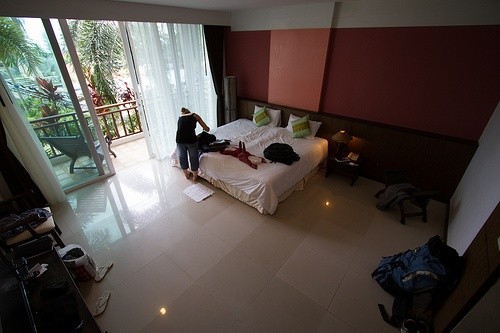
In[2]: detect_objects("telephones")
[348,151,359,161]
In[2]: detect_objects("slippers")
[94,290,110,317]
[95,262,113,282]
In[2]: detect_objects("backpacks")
[263,142,301,166]
[371,234,460,295]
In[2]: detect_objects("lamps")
[331,130,353,160]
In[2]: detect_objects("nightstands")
[325,152,364,187]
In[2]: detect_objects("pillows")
[290,114,312,138]
[252,105,281,127]
[407,154,432,178]
[284,114,322,140]
[253,106,273,127]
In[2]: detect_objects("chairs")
[0,190,65,266]
[39,133,117,175]
[375,166,445,225]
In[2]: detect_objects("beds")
[170,118,330,216]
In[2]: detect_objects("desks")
[25,251,101,333]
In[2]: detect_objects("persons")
[118,82,134,108]
[176,107,210,182]
